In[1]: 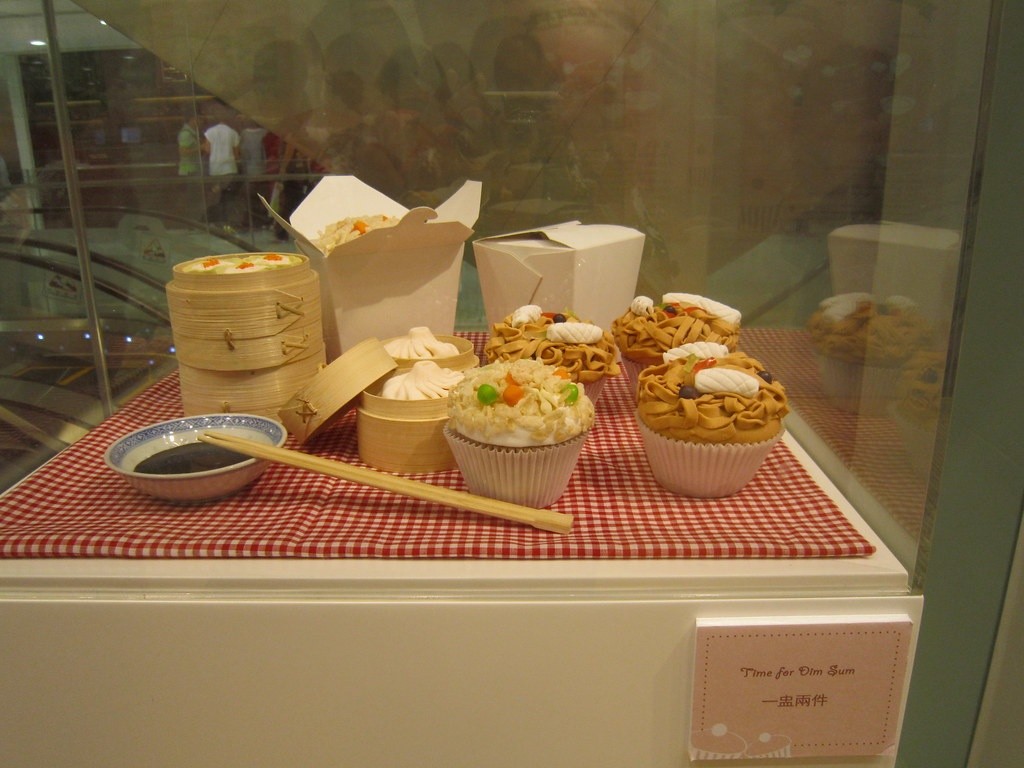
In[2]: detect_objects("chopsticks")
[198,432,575,534]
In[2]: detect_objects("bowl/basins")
[104,415,288,501]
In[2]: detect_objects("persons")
[178,109,206,230]
[273,143,325,243]
[236,119,271,233]
[204,107,240,230]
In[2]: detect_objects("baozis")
[374,327,464,400]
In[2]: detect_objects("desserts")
[447,291,791,448]
[807,292,949,478]
